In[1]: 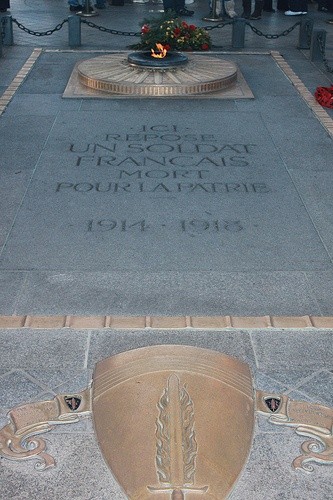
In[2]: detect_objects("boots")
[210,0,238,18]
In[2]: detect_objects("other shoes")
[109,2,124,6]
[69,4,93,11]
[327,18,333,23]
[265,8,275,12]
[319,6,328,12]
[285,10,308,15]
[241,11,262,20]
[95,4,105,9]
[164,7,194,17]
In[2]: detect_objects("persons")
[68,0,333,24]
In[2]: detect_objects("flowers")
[128,12,213,50]
